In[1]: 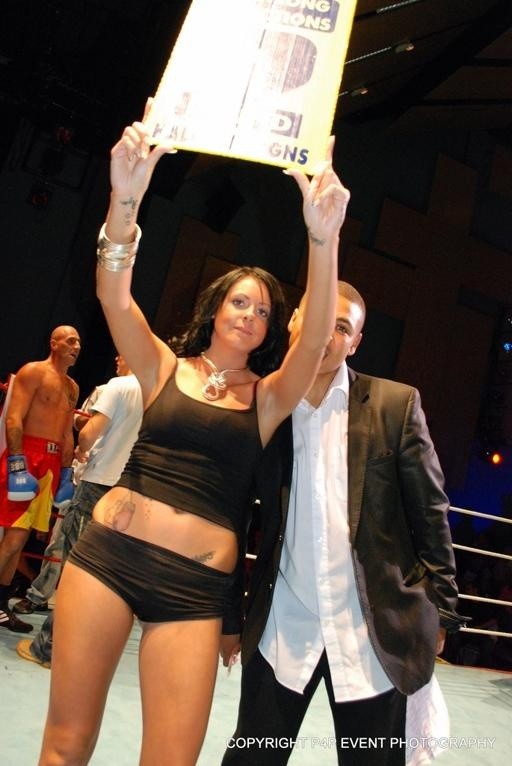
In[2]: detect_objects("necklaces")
[194,351,249,401]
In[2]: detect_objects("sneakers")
[17,640,51,669]
[0,599,48,633]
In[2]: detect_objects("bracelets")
[91,222,142,273]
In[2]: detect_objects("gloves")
[8,455,38,501]
[53,466,76,508]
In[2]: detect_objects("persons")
[37,94,351,766]
[8,382,107,615]
[17,354,143,671]
[0,325,78,635]
[216,279,471,766]
